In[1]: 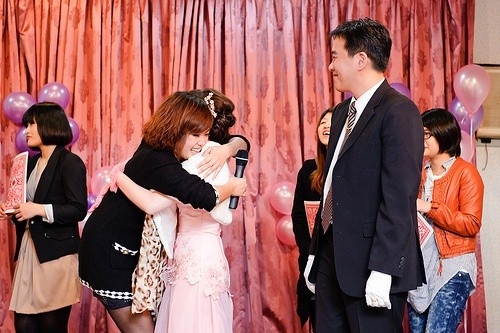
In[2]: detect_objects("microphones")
[229,149,249,210]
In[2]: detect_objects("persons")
[405,108,484,333]
[303,16,426,333]
[108,88,246,333]
[77,92,213,333]
[291,107,355,333]
[0,100,88,333]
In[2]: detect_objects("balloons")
[460,130,474,163]
[87,194,97,210]
[345,91,352,99]
[3,81,79,155]
[453,64,491,117]
[389,82,411,100]
[269,181,295,215]
[276,215,297,245]
[91,165,112,197]
[449,98,483,137]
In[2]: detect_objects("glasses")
[424,132,434,140]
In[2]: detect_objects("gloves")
[303,255,316,294]
[365,270,394,310]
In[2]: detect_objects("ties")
[321,101,357,234]
